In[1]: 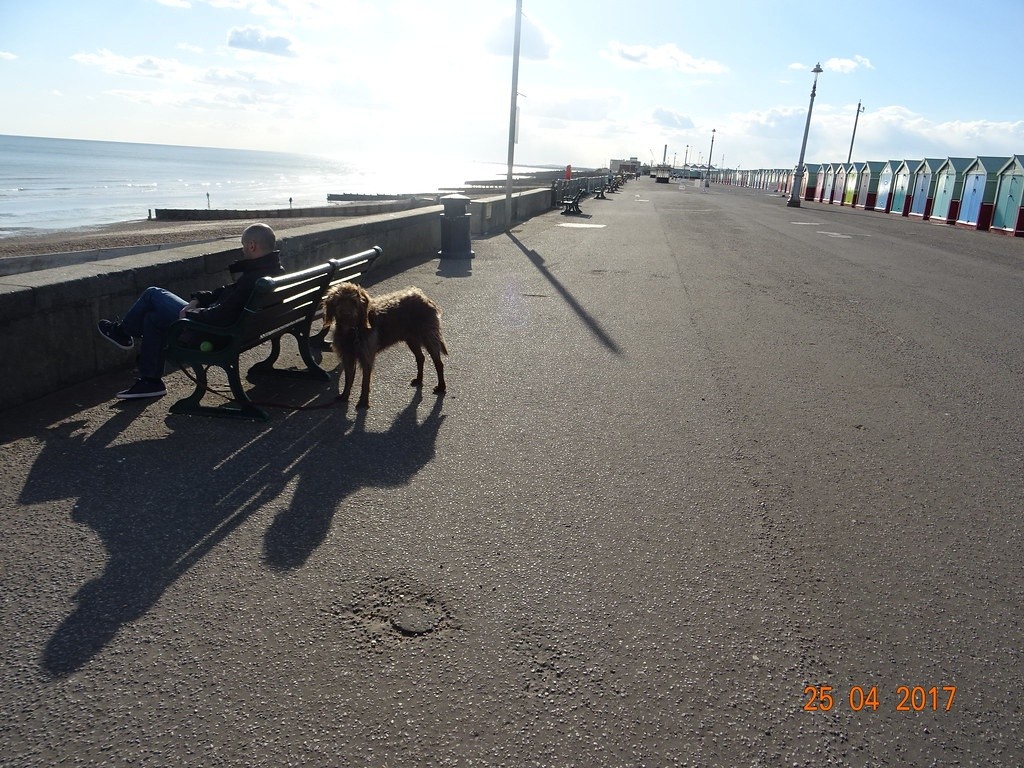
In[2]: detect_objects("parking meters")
[704,129,716,187]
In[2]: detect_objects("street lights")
[848,99,866,164]
[682,144,689,179]
[786,61,824,208]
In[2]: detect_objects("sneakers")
[98,315,134,349]
[116,379,168,398]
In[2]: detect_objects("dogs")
[323,280,451,409]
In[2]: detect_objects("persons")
[97,223,285,398]
[608,170,613,183]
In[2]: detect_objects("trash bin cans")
[436,193,475,258]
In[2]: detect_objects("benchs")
[172,247,385,422]
[592,175,631,200]
[560,188,588,215]
